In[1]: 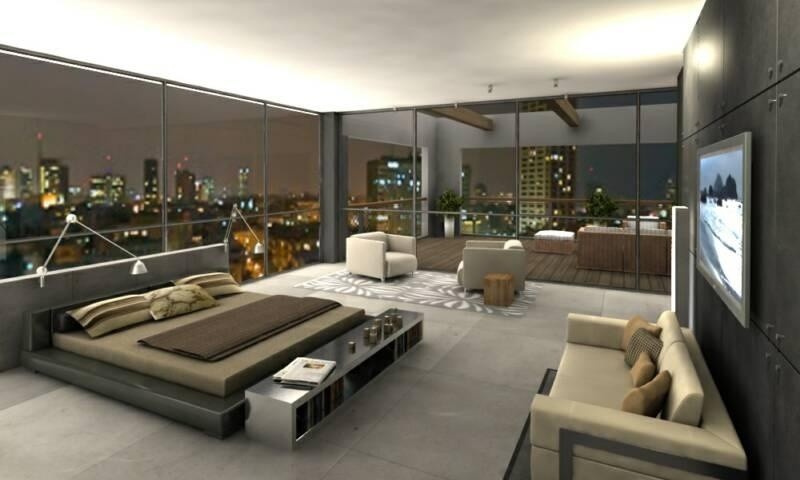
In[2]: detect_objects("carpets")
[292,269,544,318]
[503,367,558,480]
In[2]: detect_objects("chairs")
[622,215,667,230]
[456,239,526,298]
[345,231,417,282]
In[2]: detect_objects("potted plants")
[585,185,618,227]
[431,189,464,240]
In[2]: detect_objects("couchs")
[530,311,751,480]
[575,225,671,277]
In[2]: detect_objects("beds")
[19,243,366,439]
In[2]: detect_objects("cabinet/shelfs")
[244,307,424,449]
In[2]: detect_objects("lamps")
[36,214,149,276]
[223,203,265,255]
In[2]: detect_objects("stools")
[533,229,576,256]
[482,272,515,307]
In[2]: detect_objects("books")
[271,355,337,389]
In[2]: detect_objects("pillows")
[621,312,673,433]
[66,271,243,339]
